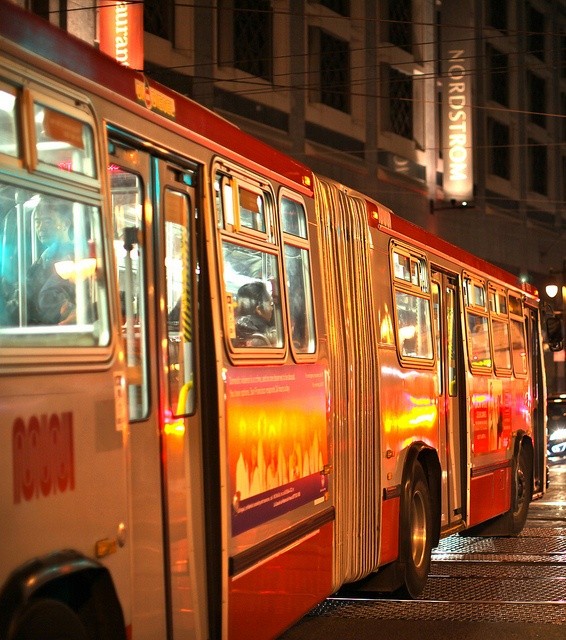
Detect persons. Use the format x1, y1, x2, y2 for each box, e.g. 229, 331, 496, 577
237, 280, 275, 347
19, 194, 76, 324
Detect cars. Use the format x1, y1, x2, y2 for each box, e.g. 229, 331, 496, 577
547, 397, 566, 466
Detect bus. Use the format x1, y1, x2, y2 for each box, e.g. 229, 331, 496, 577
1, 2, 547, 639
